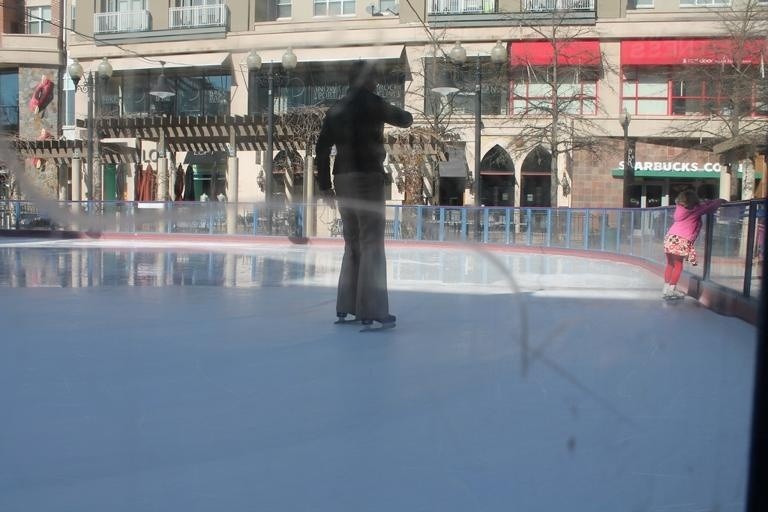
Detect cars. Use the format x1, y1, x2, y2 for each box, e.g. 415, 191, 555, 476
10, 214, 60, 231
650, 198, 747, 255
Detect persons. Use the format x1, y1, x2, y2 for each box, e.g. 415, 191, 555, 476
757, 202, 767, 278
314, 61, 414, 332
662, 188, 728, 295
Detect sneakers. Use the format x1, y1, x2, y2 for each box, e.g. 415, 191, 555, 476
337, 309, 397, 326
661, 286, 685, 299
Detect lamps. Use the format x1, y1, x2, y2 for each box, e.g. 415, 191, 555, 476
431, 54, 460, 97
149, 62, 177, 102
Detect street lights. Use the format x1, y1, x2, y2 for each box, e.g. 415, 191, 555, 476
617, 104, 635, 241
245, 46, 300, 236
447, 37, 513, 241
68, 55, 114, 215
